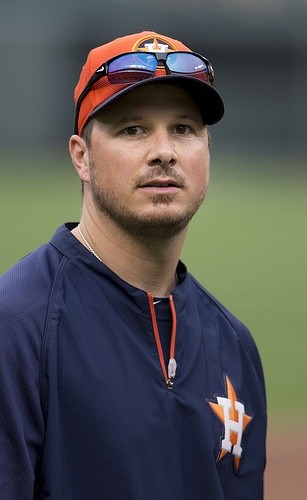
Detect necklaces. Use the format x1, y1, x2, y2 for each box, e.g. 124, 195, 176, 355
77, 223, 178, 292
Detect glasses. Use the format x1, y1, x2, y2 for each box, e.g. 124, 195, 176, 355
73, 50, 216, 135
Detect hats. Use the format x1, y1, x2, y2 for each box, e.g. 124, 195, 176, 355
73, 30, 226, 140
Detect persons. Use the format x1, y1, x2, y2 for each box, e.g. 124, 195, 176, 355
1, 31, 266, 500
